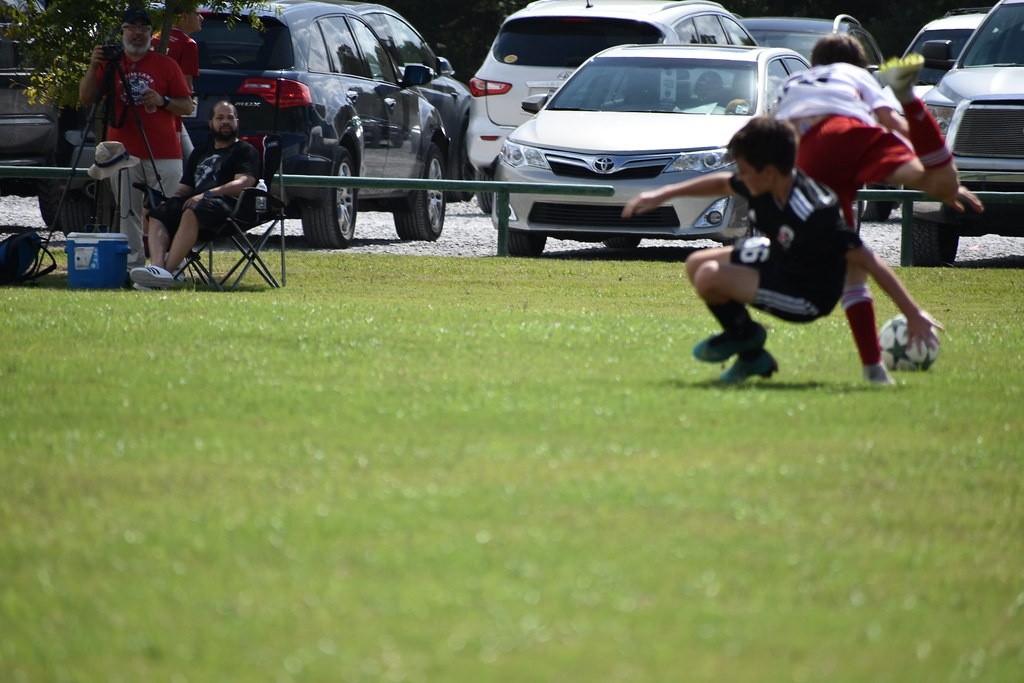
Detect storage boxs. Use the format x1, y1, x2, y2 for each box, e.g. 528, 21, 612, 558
61, 231, 129, 286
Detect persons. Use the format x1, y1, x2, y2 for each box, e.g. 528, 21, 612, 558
695, 71, 724, 103
772, 34, 983, 386
623, 118, 946, 382
79, 10, 194, 282
142, 0, 204, 255
130, 101, 260, 292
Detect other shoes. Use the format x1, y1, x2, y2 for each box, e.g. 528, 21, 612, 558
172, 266, 184, 281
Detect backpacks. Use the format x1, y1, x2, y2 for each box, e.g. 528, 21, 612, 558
0, 227, 56, 282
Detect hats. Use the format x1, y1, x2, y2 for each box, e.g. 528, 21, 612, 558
87, 141, 140, 181
122, 7, 152, 24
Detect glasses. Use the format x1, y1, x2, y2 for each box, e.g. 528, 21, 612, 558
122, 25, 151, 31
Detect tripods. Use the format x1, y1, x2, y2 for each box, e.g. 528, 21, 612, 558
28, 62, 197, 289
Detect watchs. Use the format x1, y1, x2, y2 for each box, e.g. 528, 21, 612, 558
204, 191, 211, 199
160, 95, 170, 109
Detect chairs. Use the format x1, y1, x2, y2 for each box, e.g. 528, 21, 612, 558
134, 133, 285, 292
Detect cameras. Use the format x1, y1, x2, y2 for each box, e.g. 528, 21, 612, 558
98, 45, 123, 62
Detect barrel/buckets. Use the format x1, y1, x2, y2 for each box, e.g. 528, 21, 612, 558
65, 232, 131, 289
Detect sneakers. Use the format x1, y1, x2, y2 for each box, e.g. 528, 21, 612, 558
133, 282, 166, 291
863, 364, 892, 384
721, 350, 774, 382
693, 323, 766, 361
130, 266, 174, 288
880, 53, 926, 88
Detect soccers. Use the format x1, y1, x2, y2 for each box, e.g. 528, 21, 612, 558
878, 313, 938, 370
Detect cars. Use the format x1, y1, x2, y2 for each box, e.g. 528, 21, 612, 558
492, 42, 812, 260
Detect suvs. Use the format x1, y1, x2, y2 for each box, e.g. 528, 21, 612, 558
728, 12, 885, 78
892, 0, 1024, 268
465, 1, 765, 218
0, 0, 470, 251
